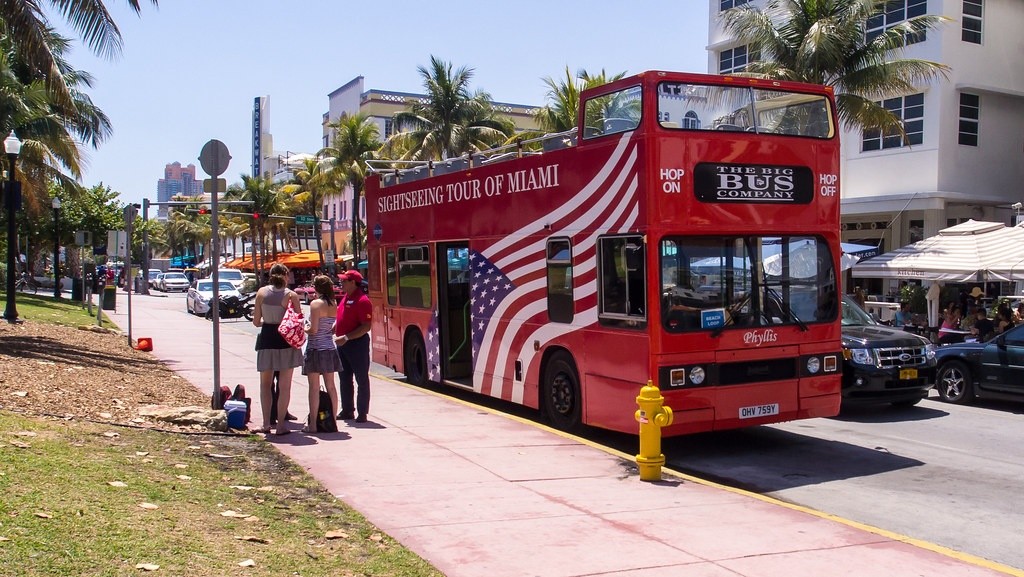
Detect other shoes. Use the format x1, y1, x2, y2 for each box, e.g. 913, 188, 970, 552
356, 414, 367, 423
276, 426, 290, 434
269, 422, 277, 428
302, 426, 317, 433
336, 411, 355, 420
285, 412, 297, 421
250, 426, 269, 433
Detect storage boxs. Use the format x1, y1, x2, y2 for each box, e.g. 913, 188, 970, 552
223, 399, 247, 430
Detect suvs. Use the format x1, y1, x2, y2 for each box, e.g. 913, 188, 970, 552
696, 284, 939, 415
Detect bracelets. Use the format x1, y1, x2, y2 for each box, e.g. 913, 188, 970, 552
344, 335, 349, 341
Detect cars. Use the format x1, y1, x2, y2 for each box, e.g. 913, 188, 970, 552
209, 269, 249, 289
139, 269, 191, 292
927, 322, 1024, 405
186, 279, 243, 316
294, 283, 344, 305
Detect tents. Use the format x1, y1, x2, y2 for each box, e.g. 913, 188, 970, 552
690, 240, 879, 279
851, 219, 1024, 326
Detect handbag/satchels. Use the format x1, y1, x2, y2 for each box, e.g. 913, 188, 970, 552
307, 384, 338, 433
212, 384, 251, 422
278, 293, 306, 349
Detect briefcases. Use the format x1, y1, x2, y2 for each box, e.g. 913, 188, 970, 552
224, 399, 247, 428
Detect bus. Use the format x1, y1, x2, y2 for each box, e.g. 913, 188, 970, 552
362, 69, 843, 433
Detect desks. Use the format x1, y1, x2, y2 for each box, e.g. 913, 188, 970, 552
938, 329, 972, 343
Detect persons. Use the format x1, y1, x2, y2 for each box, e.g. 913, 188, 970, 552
94, 266, 125, 290
251, 264, 344, 435
331, 270, 372, 422
550, 239, 643, 329
893, 281, 1024, 347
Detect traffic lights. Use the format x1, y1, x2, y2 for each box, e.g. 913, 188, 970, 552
253, 213, 268, 219
198, 210, 211, 214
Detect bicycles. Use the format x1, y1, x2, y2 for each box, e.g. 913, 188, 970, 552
14, 272, 37, 295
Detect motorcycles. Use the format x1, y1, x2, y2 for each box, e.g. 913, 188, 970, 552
205, 292, 257, 322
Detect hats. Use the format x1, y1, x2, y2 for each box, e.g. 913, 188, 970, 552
969, 287, 985, 297
337, 270, 362, 284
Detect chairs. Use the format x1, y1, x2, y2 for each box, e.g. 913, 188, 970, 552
383, 118, 773, 189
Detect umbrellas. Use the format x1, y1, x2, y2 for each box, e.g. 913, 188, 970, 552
194, 250, 343, 271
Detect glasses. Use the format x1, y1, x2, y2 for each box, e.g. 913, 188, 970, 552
339, 279, 346, 283
